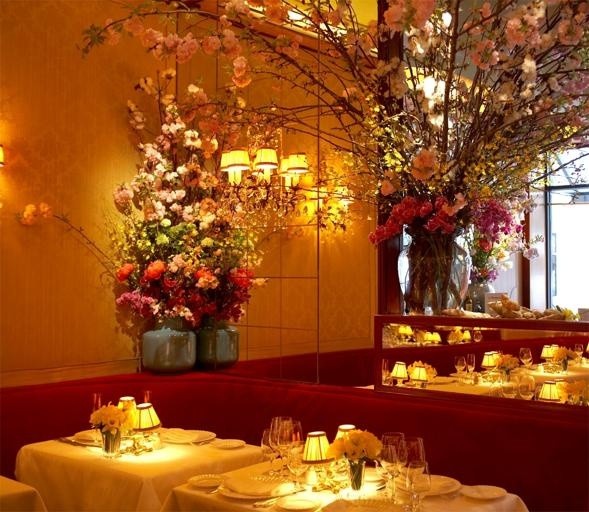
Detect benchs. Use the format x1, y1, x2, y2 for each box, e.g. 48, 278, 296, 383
0, 334, 589, 511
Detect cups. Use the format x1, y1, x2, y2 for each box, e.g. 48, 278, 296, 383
473, 331, 482, 342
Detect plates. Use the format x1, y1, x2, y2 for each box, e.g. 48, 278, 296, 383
189, 473, 405, 512
386, 474, 507, 499
432, 372, 481, 384
74, 428, 245, 449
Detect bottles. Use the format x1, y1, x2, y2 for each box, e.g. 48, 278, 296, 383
424, 307, 434, 316
404, 335, 416, 342
464, 296, 482, 312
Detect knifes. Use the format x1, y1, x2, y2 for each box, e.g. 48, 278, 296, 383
58, 438, 86, 447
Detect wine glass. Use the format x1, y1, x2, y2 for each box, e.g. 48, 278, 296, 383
490, 375, 535, 400
379, 432, 431, 512
519, 344, 584, 372
261, 417, 311, 489
456, 353, 475, 386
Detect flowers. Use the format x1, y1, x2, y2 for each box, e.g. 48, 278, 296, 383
19, 0, 589, 320
